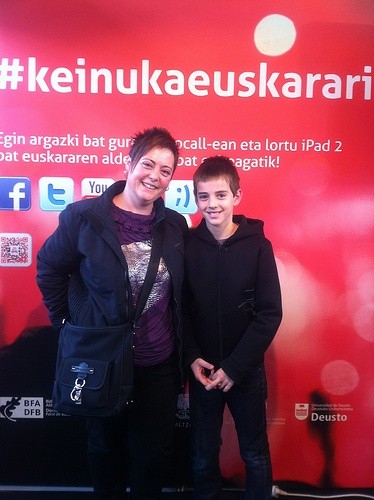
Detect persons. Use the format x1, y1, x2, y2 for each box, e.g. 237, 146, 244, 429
34, 128, 190, 500
182, 155, 283, 500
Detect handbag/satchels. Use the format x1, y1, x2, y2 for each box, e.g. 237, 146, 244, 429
53, 321, 135, 419
